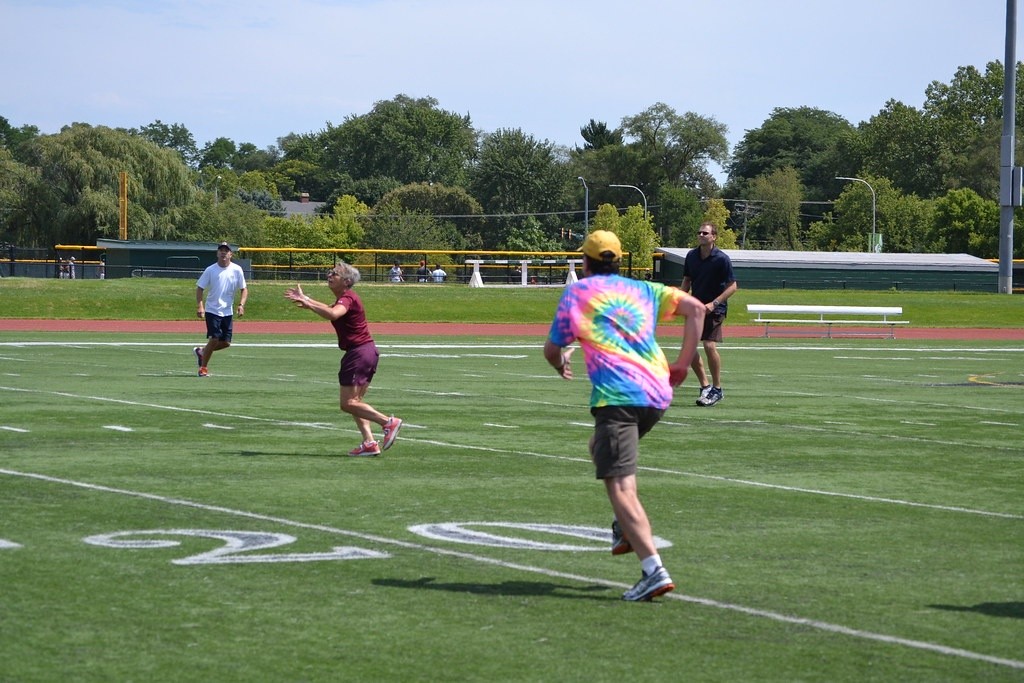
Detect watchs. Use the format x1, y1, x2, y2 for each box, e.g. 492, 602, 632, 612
713, 299, 719, 306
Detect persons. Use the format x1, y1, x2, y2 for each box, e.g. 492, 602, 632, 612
416, 260, 432, 283
511, 267, 522, 284
96, 259, 105, 279
55, 257, 76, 279
432, 264, 447, 283
544, 230, 706, 601
645, 273, 652, 281
388, 260, 406, 283
562, 268, 578, 285
680, 223, 738, 406
531, 277, 537, 285
283, 262, 403, 456
193, 242, 248, 378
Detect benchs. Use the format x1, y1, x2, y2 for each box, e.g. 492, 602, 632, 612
747, 304, 909, 340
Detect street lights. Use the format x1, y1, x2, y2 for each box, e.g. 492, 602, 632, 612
835, 177, 875, 253
215, 175, 225, 197
609, 185, 647, 220
577, 177, 590, 235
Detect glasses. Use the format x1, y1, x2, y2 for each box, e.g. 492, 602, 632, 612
697, 231, 713, 236
326, 269, 344, 278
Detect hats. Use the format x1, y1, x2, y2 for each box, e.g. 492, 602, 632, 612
216, 241, 232, 250
583, 231, 623, 263
420, 260, 424, 265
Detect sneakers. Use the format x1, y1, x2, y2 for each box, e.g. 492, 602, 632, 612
622, 566, 675, 602
347, 441, 381, 457
697, 384, 712, 403
198, 367, 208, 377
612, 520, 633, 555
195, 347, 204, 369
700, 387, 723, 406
382, 414, 402, 450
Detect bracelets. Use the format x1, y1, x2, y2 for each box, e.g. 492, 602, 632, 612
305, 296, 309, 301
555, 354, 566, 370
238, 304, 244, 307
